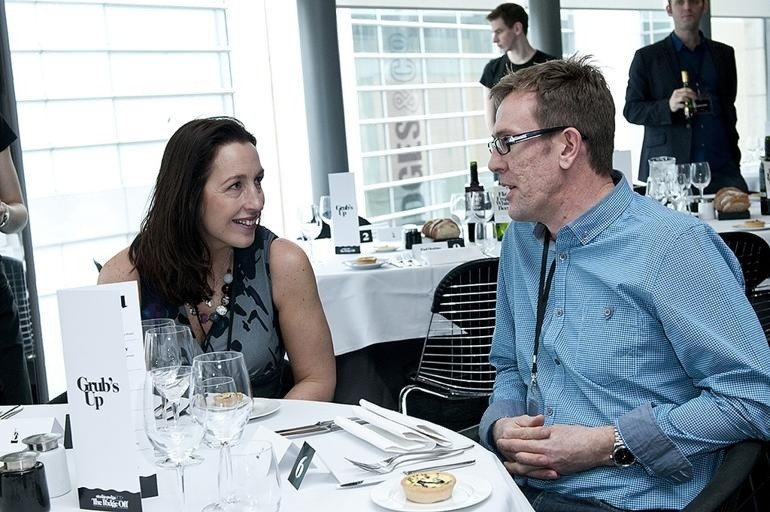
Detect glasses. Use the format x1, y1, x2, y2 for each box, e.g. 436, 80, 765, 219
485, 124, 574, 155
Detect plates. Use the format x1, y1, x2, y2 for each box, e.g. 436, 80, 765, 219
341, 224, 465, 270
729, 222, 770, 232
370, 473, 493, 512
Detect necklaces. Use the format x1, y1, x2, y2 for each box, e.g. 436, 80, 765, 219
189, 252, 234, 320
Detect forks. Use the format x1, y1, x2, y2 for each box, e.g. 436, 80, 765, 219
358, 450, 464, 473
344, 444, 479, 468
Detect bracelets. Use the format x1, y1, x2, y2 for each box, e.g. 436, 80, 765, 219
0, 204, 10, 230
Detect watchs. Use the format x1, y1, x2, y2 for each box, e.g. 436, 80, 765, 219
610, 426, 636, 470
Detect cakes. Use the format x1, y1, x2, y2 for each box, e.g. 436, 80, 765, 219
356, 255, 376, 265
213, 393, 243, 407
746, 220, 765, 228
401, 471, 456, 505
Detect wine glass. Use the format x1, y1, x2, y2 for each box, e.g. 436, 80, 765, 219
135, 315, 282, 512
319, 196, 333, 245
450, 159, 509, 259
297, 203, 322, 259
644, 155, 713, 223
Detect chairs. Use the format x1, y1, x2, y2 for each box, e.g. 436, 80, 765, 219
674, 436, 769, 510
306, 212, 372, 238
396, 250, 501, 432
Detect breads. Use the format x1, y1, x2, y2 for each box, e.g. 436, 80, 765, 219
422, 218, 461, 239
714, 186, 751, 213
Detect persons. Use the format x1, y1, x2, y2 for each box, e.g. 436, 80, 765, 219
622, 0, 748, 196
478, 4, 561, 130
478, 51, 769, 511
0, 114, 33, 404
97, 116, 338, 408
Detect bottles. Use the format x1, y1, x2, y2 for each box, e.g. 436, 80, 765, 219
18, 431, 73, 500
757, 135, 770, 217
697, 198, 716, 222
680, 70, 704, 131
0, 450, 52, 511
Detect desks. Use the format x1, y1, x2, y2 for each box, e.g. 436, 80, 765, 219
273, 219, 770, 362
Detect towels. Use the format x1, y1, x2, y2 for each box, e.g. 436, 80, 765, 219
334, 398, 454, 455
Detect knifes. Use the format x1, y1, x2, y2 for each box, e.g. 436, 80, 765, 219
335, 460, 477, 491
279, 421, 369, 438
271, 416, 360, 434
